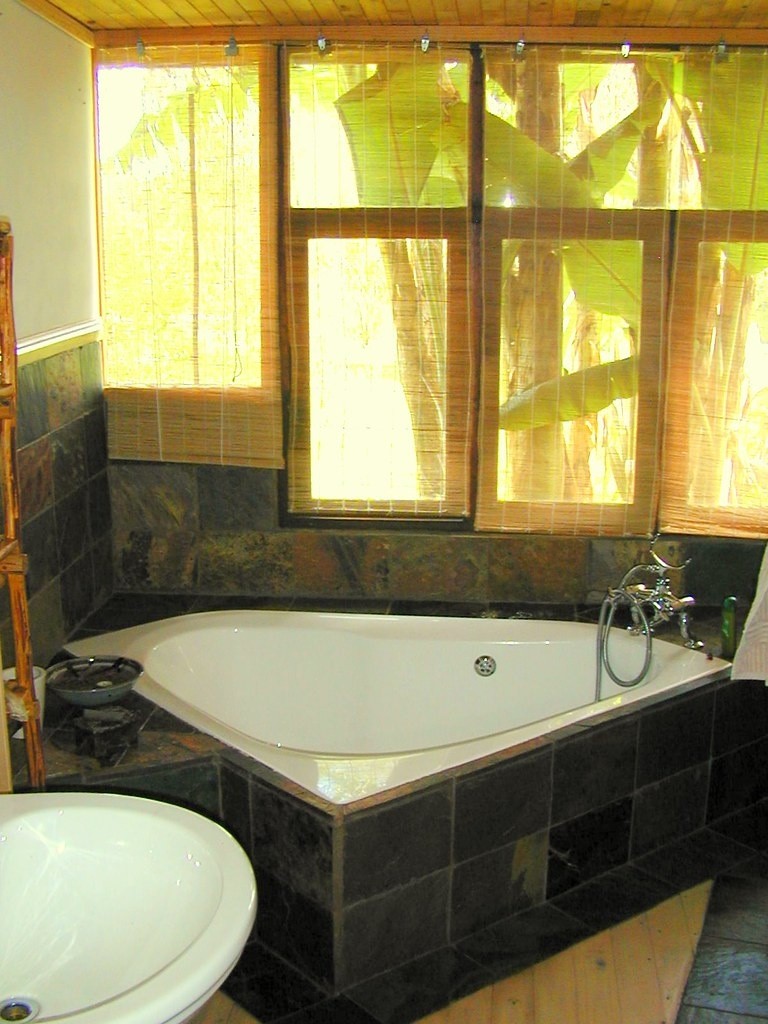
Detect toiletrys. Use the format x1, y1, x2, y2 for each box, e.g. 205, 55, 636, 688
720, 595, 737, 658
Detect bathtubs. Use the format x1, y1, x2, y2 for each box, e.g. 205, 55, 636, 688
58, 603, 740, 809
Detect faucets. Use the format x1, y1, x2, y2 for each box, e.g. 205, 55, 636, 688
626, 614, 662, 637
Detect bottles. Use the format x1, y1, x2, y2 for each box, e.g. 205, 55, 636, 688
720, 596, 738, 658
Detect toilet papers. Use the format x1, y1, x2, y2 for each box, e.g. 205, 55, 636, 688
3, 664, 48, 739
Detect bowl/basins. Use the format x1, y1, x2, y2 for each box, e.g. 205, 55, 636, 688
45, 654, 145, 705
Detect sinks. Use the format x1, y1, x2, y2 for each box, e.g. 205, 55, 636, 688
0, 788, 257, 1023
